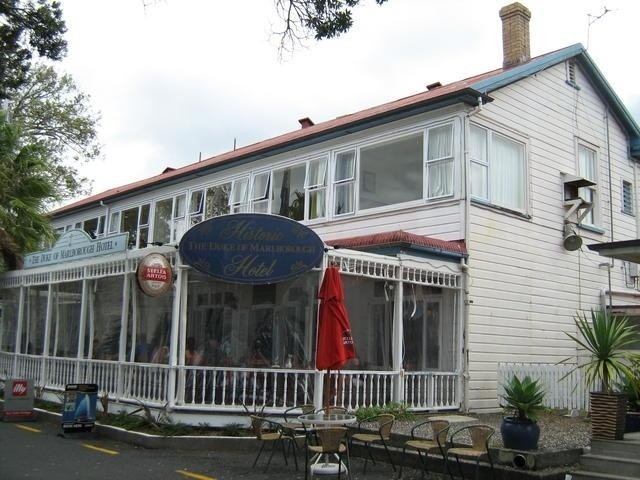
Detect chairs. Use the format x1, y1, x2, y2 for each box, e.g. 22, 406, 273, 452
315, 406, 347, 415
351, 414, 398, 474
304, 426, 354, 480
249, 414, 298, 474
281, 404, 317, 459
399, 418, 455, 479
445, 423, 498, 479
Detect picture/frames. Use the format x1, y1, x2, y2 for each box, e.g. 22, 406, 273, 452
362, 170, 377, 193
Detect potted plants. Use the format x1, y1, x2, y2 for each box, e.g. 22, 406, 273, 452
498, 373, 547, 451
553, 300, 639, 444
610, 358, 639, 433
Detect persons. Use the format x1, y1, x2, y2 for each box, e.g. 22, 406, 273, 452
136, 334, 152, 362
186, 337, 202, 365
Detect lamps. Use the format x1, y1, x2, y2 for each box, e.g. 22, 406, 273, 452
561, 220, 583, 252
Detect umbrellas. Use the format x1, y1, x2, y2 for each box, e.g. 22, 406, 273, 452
308, 264, 357, 467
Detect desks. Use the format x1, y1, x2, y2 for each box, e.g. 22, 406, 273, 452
298, 412, 357, 476
